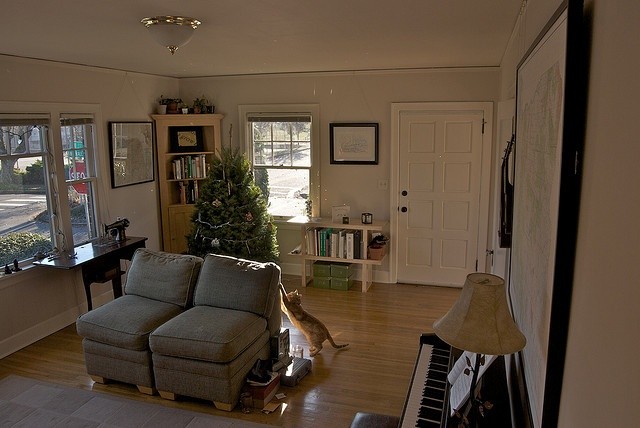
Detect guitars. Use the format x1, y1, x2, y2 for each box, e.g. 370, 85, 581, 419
498, 134, 515, 248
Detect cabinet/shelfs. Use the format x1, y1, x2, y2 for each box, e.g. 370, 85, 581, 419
284, 211, 390, 294
150, 113, 225, 253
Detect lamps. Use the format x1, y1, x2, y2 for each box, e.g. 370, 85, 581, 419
139, 14, 202, 58
432, 272, 527, 422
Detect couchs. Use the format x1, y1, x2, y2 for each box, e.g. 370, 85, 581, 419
148, 252, 281, 412
75, 247, 204, 396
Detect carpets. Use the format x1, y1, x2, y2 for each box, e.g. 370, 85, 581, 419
0, 373, 278, 428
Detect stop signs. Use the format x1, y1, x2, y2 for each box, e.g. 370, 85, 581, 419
69, 161, 89, 195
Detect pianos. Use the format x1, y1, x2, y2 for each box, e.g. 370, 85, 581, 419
397, 333, 526, 428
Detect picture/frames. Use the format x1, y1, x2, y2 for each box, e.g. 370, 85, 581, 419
107, 121, 155, 189
329, 122, 379, 165
505, 0, 591, 428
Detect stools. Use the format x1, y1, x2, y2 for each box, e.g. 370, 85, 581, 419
347, 411, 400, 428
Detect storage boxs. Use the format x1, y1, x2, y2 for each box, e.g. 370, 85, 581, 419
331, 272, 356, 291
241, 372, 281, 410
330, 264, 352, 278
313, 276, 331, 289
312, 260, 331, 277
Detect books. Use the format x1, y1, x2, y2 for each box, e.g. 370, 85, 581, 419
171, 155, 211, 179
305, 229, 363, 260
179, 181, 198, 205
447, 351, 498, 417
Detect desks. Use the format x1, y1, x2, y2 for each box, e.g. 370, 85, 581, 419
31, 236, 148, 269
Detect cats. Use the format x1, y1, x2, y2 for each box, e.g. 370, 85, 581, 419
279, 283, 349, 357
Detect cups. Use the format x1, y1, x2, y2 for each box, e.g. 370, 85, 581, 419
240, 392, 253, 414
292, 345, 303, 358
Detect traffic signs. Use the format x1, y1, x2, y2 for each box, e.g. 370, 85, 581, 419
69, 141, 85, 156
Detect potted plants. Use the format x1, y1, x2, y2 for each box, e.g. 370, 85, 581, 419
373, 234, 390, 254
190, 96, 209, 114
179, 102, 189, 114
368, 239, 383, 259
158, 97, 183, 114
157, 94, 167, 115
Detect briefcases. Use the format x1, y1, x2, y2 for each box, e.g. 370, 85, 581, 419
279, 355, 312, 385
